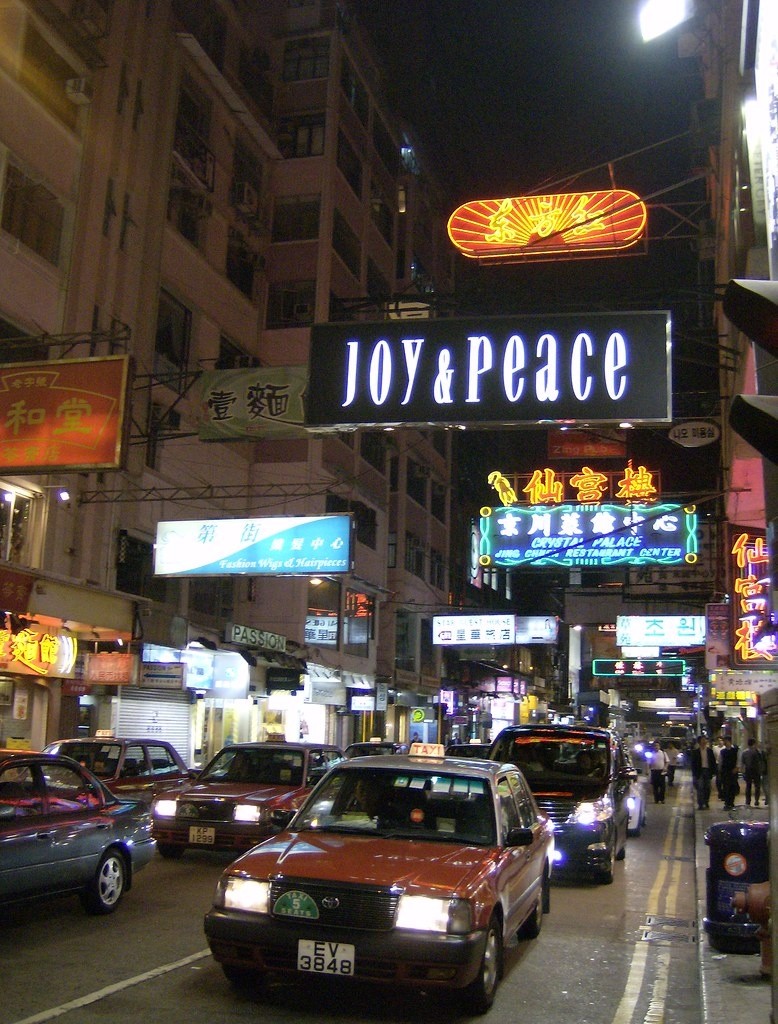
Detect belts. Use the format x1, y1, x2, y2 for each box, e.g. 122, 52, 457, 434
651, 770, 662, 771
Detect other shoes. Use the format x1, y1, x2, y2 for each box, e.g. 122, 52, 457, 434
746, 801, 750, 805
765, 801, 768, 805
722, 805, 731, 811
655, 801, 658, 804
754, 801, 759, 806
705, 803, 709, 808
718, 794, 722, 800
661, 801, 665, 804
698, 804, 703, 810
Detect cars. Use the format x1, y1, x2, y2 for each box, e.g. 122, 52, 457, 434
202, 742, 557, 1017
313, 737, 411, 789
653, 738, 688, 768
445, 739, 497, 763
148, 732, 350, 859
13, 729, 189, 819
0, 750, 160, 918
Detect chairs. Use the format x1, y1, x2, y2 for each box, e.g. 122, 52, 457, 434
456, 801, 491, 832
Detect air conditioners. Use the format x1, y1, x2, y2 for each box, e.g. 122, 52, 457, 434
64, 77, 95, 105
294, 303, 314, 319
233, 182, 258, 216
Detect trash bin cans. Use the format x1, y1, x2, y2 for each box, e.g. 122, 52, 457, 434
703, 818, 772, 955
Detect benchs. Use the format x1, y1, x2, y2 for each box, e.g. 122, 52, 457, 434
114, 758, 165, 769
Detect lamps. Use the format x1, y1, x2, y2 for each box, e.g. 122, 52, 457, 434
43, 485, 70, 500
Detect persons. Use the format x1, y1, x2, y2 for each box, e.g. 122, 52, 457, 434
92, 760, 106, 771
692, 735, 769, 811
410, 732, 423, 748
649, 740, 678, 804
575, 750, 597, 777
348, 779, 375, 812
440, 733, 471, 746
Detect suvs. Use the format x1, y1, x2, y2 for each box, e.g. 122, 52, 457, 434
480, 723, 642, 884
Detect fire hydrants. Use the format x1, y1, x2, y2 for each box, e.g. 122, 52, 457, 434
731, 880, 774, 980
